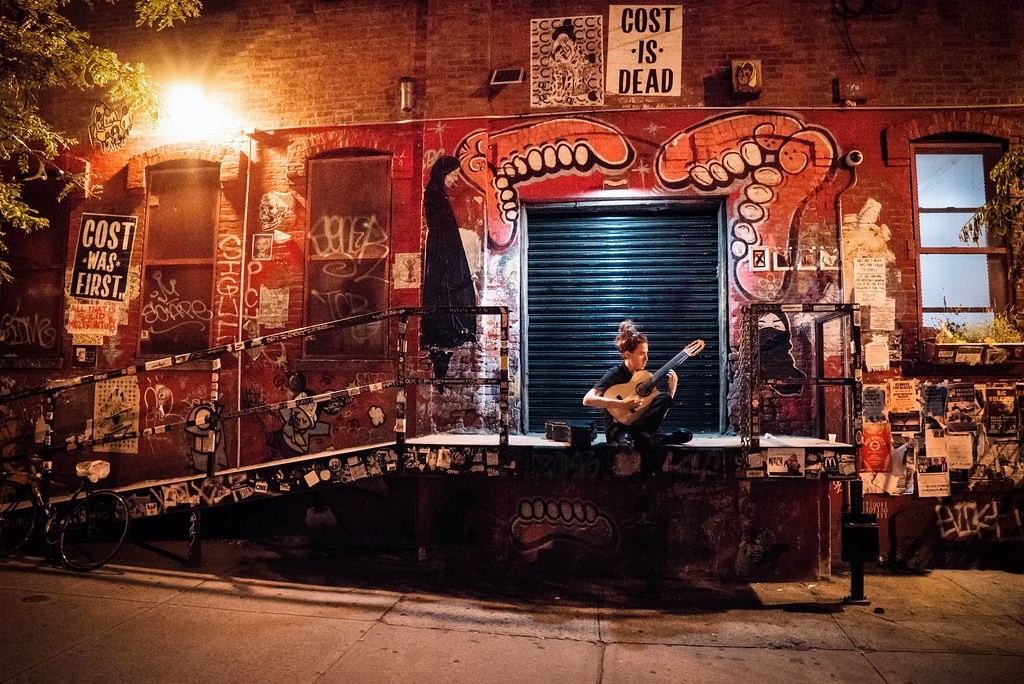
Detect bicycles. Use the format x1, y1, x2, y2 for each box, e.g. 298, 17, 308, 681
0, 455, 130, 570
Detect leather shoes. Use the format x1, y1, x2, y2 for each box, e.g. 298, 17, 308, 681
615, 432, 635, 453
654, 427, 694, 445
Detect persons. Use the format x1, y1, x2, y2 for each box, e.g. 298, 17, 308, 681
582, 319, 693, 453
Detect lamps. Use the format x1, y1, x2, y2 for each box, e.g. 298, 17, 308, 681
400, 76, 416, 113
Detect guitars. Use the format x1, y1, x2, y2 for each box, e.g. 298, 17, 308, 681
601, 338, 706, 425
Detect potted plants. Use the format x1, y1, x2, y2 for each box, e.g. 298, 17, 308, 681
919, 296, 1024, 365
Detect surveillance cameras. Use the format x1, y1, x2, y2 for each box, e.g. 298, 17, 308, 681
846, 150, 863, 167
